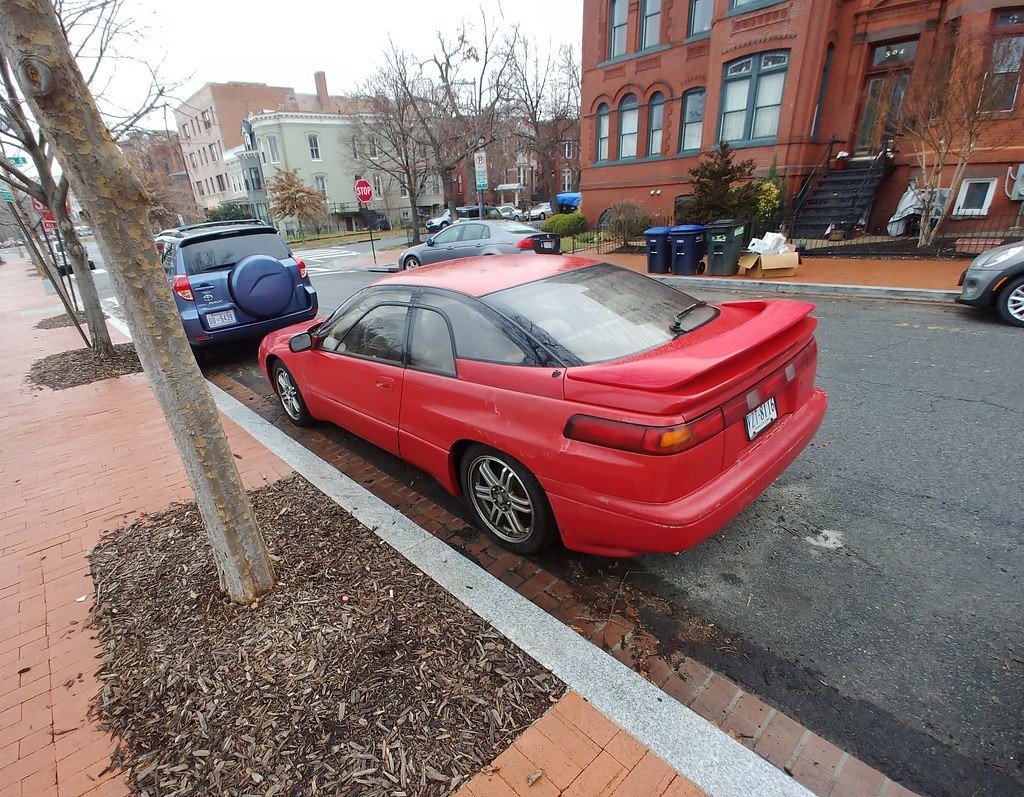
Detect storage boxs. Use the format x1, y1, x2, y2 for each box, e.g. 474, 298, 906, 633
737, 244, 802, 279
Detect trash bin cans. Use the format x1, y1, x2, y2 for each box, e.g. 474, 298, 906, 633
670, 224, 706, 276
704, 219, 747, 276
643, 226, 671, 274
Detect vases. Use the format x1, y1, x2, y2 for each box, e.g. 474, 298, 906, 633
829, 230, 845, 241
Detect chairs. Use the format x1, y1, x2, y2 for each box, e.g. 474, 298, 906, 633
421, 303, 473, 368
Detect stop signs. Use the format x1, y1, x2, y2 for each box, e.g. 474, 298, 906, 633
354, 180, 373, 201
31, 192, 71, 221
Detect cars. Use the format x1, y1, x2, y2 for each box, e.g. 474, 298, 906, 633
48, 240, 96, 277
398, 219, 563, 270
258, 253, 828, 556
955, 238, 1024, 328
490, 206, 523, 222
425, 207, 469, 230
523, 203, 554, 221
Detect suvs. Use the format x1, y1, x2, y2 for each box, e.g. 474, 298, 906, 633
153, 219, 319, 347
